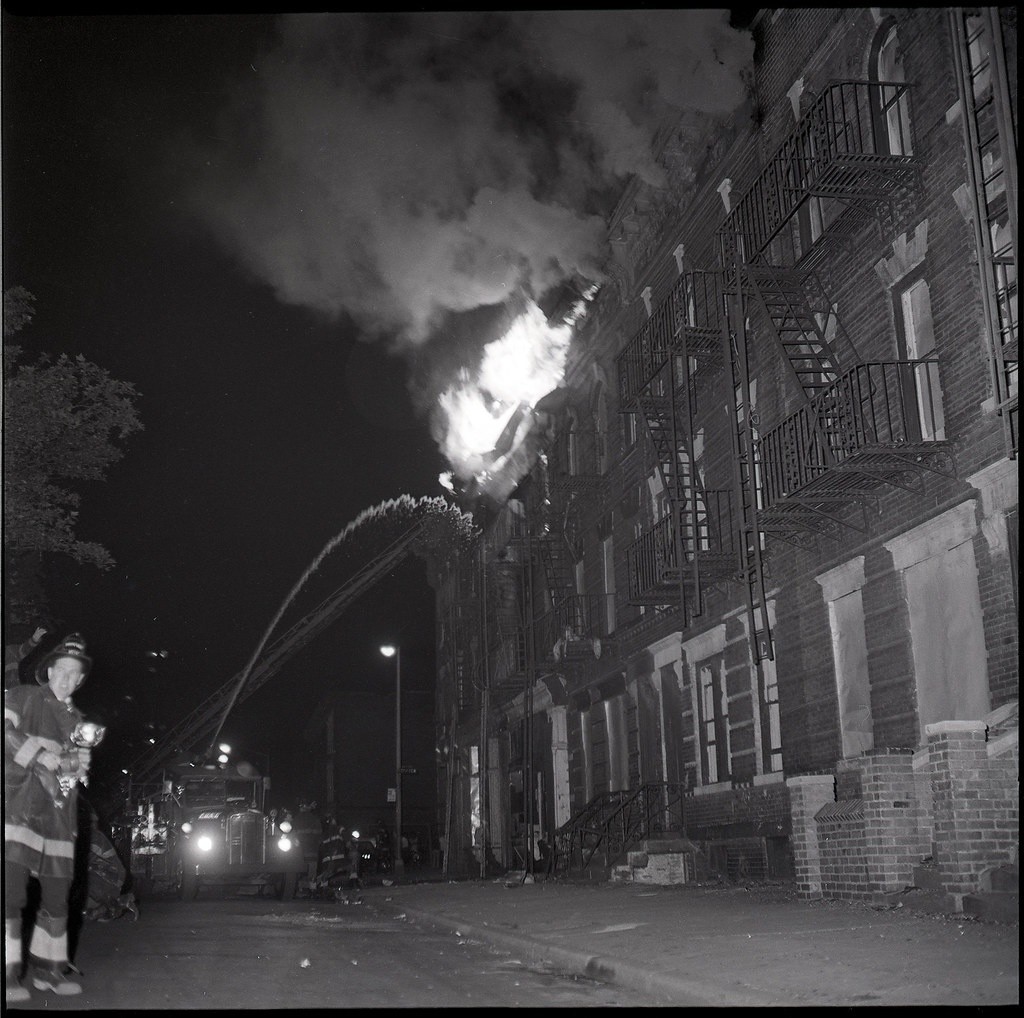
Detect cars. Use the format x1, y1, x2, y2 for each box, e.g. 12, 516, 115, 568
353, 838, 407, 886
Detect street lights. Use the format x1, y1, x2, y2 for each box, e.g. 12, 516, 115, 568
122, 769, 132, 810
380, 635, 401, 865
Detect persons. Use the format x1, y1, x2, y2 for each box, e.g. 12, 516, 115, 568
359, 824, 419, 875
273, 800, 365, 903
5, 627, 139, 1001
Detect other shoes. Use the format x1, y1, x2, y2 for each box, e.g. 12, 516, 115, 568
6, 967, 83, 1000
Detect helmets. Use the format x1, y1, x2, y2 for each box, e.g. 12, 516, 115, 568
324, 814, 335, 824
277, 807, 290, 821
36, 632, 93, 689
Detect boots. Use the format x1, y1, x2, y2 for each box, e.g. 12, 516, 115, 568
351, 875, 360, 891
321, 876, 329, 887
308, 877, 318, 890
97, 890, 141, 924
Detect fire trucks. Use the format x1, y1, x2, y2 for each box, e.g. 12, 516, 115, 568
111, 742, 307, 903
